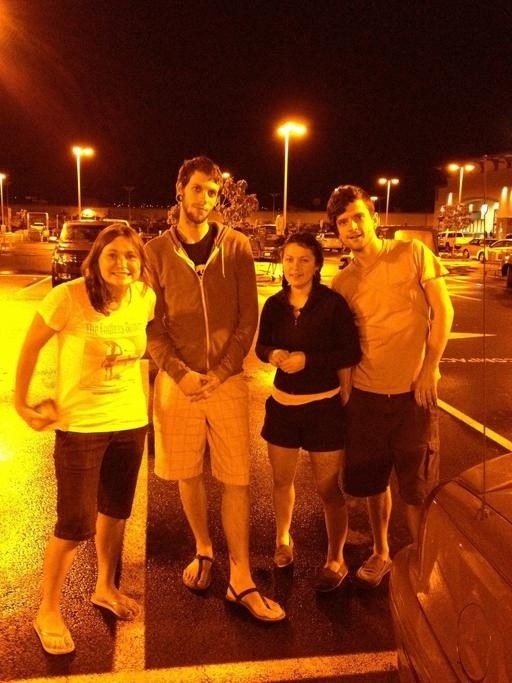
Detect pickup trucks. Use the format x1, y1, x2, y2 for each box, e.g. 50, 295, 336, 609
437, 230, 473, 250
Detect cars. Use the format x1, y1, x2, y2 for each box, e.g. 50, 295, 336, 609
500, 254, 512, 286
315, 232, 347, 251
460, 238, 497, 259
250, 223, 284, 262
388, 450, 510, 682
31, 223, 45, 230
503, 233, 512, 239
476, 238, 512, 262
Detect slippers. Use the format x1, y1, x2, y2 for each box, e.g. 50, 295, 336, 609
90, 594, 141, 621
32, 619, 75, 655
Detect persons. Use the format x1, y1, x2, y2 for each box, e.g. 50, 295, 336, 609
145, 155, 288, 624
252, 232, 356, 594
326, 183, 459, 586
12, 220, 160, 655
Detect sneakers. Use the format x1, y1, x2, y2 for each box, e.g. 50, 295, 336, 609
274, 532, 294, 569
356, 554, 392, 586
312, 561, 348, 592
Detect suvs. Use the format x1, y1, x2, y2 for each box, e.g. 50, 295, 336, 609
48, 218, 130, 290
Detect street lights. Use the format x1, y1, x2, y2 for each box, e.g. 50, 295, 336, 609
447, 162, 476, 207
0, 173, 7, 224
72, 146, 95, 215
274, 121, 307, 230
377, 176, 402, 225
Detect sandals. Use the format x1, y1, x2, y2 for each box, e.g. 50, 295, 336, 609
183, 554, 214, 591
224, 582, 286, 622
